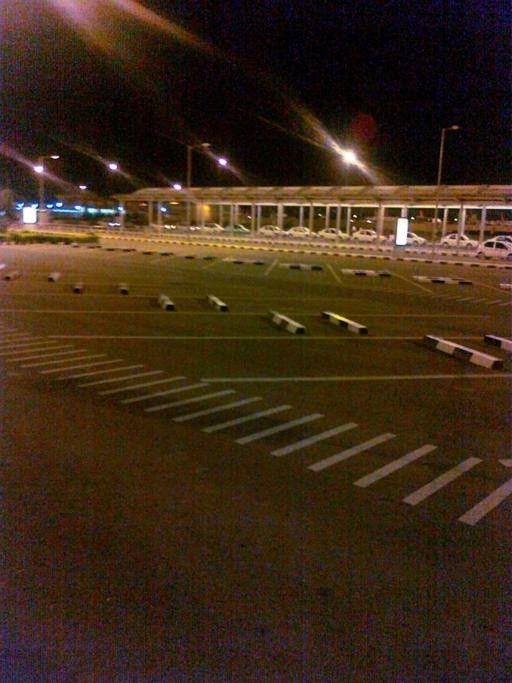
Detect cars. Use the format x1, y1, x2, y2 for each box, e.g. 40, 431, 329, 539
286, 226, 317, 241
159, 223, 177, 232
259, 225, 287, 238
352, 228, 388, 244
203, 223, 223, 231
440, 232, 480, 250
105, 220, 120, 229
317, 227, 350, 242
388, 231, 428, 248
476, 234, 512, 260
188, 224, 202, 232
224, 223, 250, 234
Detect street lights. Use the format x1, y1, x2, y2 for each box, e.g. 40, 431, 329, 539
183, 142, 211, 226
434, 125, 460, 222
37, 154, 60, 220
